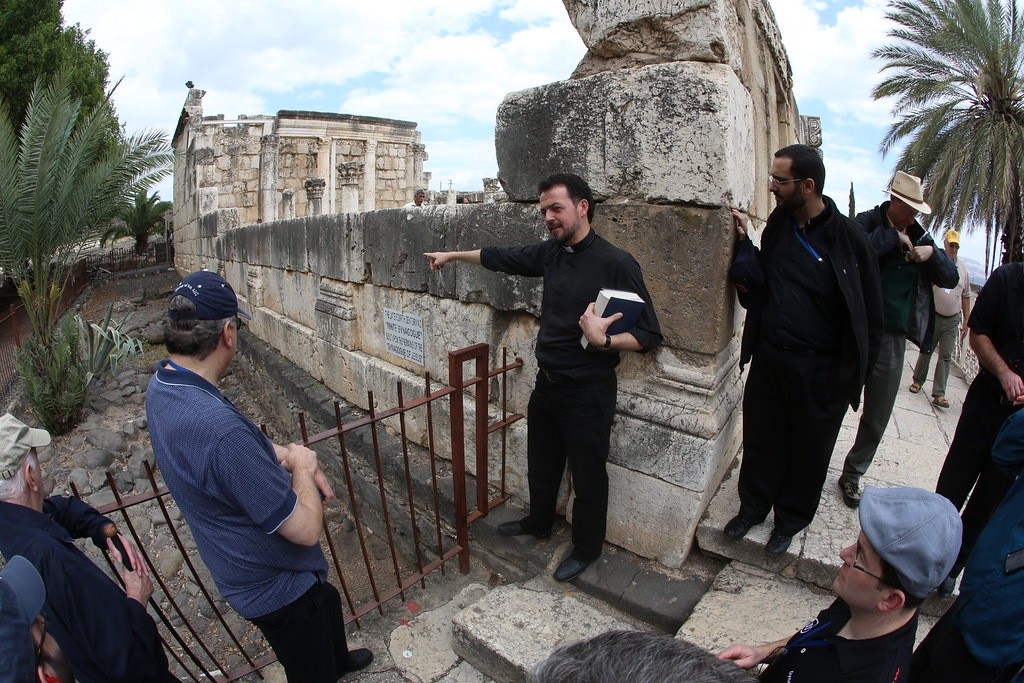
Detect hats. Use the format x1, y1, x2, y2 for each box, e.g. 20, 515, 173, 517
859, 485, 963, 599
945, 230, 960, 245
0, 413, 51, 481
0, 555, 46, 683
882, 170, 932, 215
168, 269, 253, 321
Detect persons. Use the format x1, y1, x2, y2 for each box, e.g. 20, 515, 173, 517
721, 144, 885, 558
715, 484, 964, 683
147, 272, 373, 682
936, 262, 1024, 598
525, 628, 759, 683
405, 189, 425, 207
908, 228, 971, 408
838, 168, 959, 508
420, 172, 663, 582
907, 408, 1024, 683
0, 555, 77, 683
0, 413, 185, 683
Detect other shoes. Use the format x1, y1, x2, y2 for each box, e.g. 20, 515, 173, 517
838, 475, 860, 508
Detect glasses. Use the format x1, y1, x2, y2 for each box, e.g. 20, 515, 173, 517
746, 646, 789, 681
768, 175, 803, 187
229, 318, 246, 331
853, 538, 910, 609
946, 240, 959, 249
36, 610, 48, 670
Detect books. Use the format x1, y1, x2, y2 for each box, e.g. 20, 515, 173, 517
580, 288, 646, 351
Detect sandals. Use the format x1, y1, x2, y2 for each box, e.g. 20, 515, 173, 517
932, 395, 949, 408
909, 382, 922, 393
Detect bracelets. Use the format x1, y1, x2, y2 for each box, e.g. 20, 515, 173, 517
603, 335, 611, 350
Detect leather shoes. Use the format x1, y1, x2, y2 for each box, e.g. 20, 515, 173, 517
764, 529, 793, 559
552, 555, 593, 582
496, 520, 527, 535
938, 575, 956, 599
723, 515, 761, 540
336, 648, 374, 679
